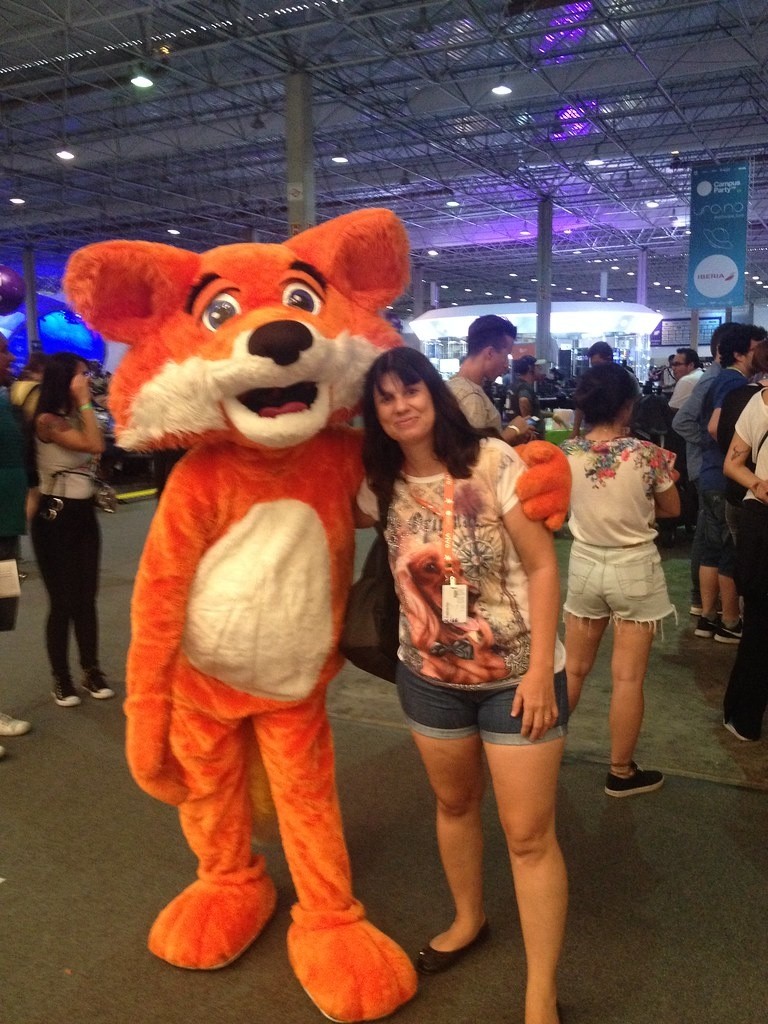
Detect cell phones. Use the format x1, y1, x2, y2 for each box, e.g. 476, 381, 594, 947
527, 416, 541, 424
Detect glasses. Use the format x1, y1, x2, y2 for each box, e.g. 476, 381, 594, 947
670, 361, 688, 366
74, 371, 95, 377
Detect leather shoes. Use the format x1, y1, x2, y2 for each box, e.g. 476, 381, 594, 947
413, 909, 488, 975
556, 999, 563, 1024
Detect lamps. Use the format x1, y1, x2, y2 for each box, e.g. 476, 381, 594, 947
6, 6, 681, 255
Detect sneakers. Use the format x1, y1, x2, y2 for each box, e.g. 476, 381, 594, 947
690, 615, 743, 644
81, 667, 115, 699
0, 711, 31, 737
50, 673, 82, 707
604, 760, 665, 798
0, 746, 5, 757
689, 595, 741, 615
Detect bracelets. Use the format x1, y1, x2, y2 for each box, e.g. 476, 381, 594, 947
509, 424, 521, 435
77, 401, 94, 412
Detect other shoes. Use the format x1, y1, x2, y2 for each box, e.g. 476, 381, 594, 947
722, 714, 753, 742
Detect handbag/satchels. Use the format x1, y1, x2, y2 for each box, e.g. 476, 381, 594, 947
725, 430, 768, 507
12, 383, 43, 427
338, 476, 401, 685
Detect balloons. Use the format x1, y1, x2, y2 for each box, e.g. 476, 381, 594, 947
0, 264, 27, 316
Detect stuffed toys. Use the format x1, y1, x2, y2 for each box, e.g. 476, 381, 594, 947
60, 205, 574, 1024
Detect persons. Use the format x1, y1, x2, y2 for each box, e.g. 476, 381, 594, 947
0, 313, 768, 760
353, 345, 570, 1024
561, 362, 681, 796
